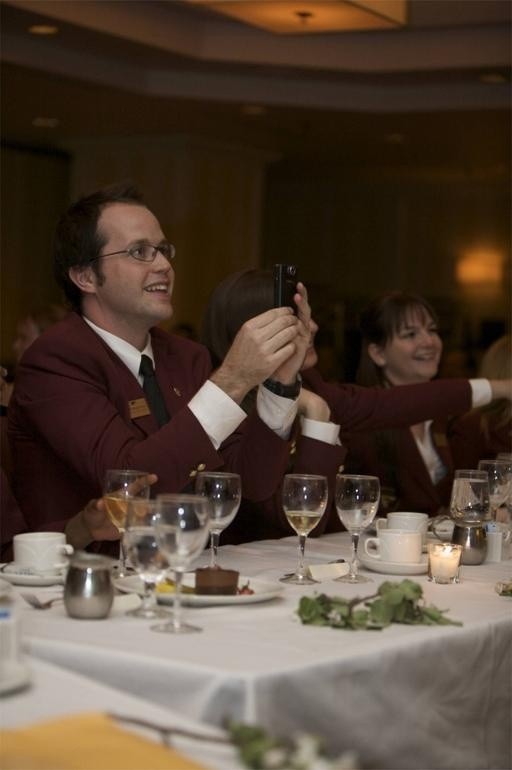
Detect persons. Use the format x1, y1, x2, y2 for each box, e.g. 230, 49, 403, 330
0, 303, 71, 466
0, 464, 159, 564
206, 267, 512, 548
172, 323, 198, 342
450, 333, 512, 468
327, 290, 512, 534
8, 186, 311, 565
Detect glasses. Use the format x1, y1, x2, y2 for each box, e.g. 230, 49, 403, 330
83, 241, 178, 264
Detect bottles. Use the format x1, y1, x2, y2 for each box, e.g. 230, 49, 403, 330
62, 552, 114, 621
450, 469, 492, 525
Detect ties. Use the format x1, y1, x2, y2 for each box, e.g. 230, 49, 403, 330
138, 353, 171, 430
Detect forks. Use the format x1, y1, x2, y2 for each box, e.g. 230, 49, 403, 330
19, 591, 65, 611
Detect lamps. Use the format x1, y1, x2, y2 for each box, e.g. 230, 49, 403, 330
211, 0, 408, 36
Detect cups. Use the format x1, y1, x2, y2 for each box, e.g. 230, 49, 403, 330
376, 511, 428, 546
1, 610, 18, 670
362, 527, 425, 565
13, 532, 75, 575
424, 540, 464, 585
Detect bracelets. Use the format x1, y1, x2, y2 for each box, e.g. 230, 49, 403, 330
263, 374, 302, 398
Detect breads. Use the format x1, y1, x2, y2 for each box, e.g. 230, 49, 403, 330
195, 568, 240, 596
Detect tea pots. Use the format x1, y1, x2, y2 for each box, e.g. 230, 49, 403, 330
432, 516, 487, 566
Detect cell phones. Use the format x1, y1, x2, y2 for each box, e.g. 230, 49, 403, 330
275, 263, 299, 313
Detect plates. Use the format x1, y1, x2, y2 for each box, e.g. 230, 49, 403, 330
356, 554, 432, 577
109, 569, 293, 607
0, 662, 38, 698
422, 537, 444, 554
0, 562, 70, 587
425, 516, 454, 534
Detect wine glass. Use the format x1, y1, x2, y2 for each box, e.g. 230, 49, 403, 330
494, 453, 511, 544
102, 464, 210, 633
331, 472, 382, 584
478, 459, 512, 534
192, 471, 243, 569
277, 472, 328, 586
